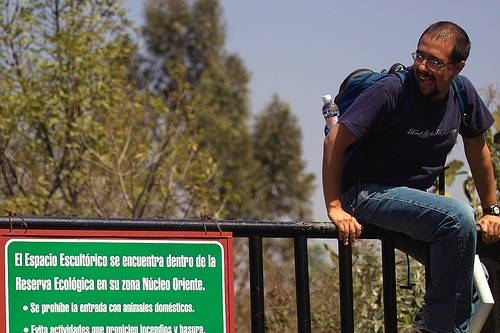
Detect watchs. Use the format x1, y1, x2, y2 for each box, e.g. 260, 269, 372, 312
481, 203, 500, 216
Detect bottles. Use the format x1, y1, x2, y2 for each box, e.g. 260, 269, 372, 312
322, 95, 340, 130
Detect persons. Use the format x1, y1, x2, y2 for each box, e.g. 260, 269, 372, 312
321, 21, 500, 333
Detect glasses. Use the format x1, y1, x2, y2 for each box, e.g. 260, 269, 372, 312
410, 52, 454, 68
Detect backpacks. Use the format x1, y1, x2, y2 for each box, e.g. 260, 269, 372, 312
324, 64, 471, 138
